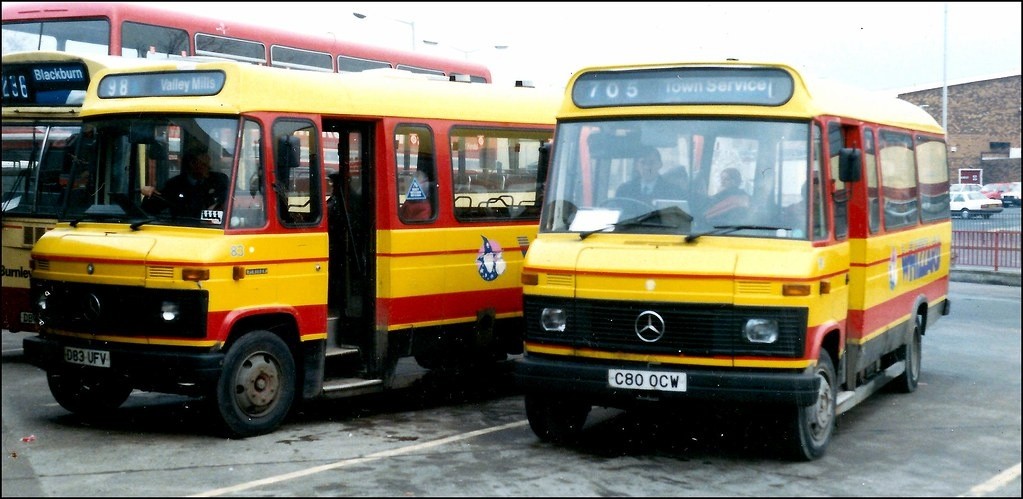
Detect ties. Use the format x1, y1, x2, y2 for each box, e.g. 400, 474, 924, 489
642, 187, 648, 197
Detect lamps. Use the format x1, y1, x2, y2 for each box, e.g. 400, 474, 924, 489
951, 144, 959, 153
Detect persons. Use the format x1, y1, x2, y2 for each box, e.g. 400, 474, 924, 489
142, 143, 229, 223
709, 168, 752, 205
612, 147, 687, 226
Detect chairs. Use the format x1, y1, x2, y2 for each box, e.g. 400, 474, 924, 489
455, 194, 538, 219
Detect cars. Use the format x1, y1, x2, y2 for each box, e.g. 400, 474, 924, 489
978, 183, 1017, 200
921, 191, 1004, 220
950, 184, 982, 191
1001, 184, 1021, 208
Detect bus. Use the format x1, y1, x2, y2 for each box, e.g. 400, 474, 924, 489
0, 55, 546, 334
21, 60, 704, 441
510, 60, 952, 463
1, 2, 498, 201
